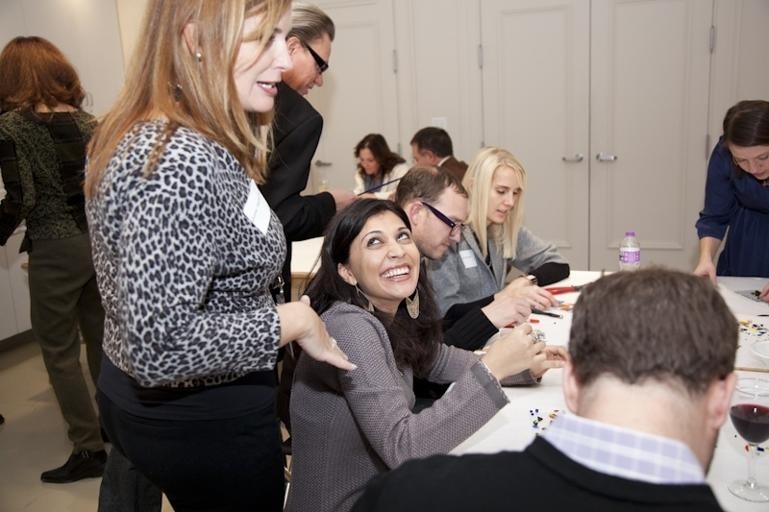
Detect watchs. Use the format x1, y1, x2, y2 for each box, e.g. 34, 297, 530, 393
525, 274, 541, 286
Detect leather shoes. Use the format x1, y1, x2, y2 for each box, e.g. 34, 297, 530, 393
40, 450, 108, 484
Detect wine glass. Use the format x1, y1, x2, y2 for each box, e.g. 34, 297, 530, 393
729, 377, 768, 504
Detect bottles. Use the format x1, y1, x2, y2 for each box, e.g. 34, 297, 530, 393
619, 230, 642, 274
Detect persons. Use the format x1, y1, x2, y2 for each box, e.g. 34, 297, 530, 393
79, 0, 297, 512
424, 145, 571, 333
352, 134, 406, 195
348, 260, 740, 511
276, 162, 532, 440
1, 34, 112, 483
409, 126, 468, 185
276, 195, 572, 511
690, 99, 769, 303
97, 1, 394, 511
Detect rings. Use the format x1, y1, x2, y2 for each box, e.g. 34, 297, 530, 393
531, 335, 539, 344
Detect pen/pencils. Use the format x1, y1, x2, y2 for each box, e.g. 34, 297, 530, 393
734, 367, 769, 372
532, 309, 560, 318
757, 315, 769, 316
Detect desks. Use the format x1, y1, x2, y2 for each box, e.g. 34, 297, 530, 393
445, 270, 769, 511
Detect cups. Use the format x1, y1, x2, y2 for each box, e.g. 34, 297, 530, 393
310, 164, 333, 195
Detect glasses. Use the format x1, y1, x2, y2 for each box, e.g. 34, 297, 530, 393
302, 39, 329, 75
422, 200, 465, 237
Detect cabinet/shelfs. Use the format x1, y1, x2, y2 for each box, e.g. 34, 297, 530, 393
292, 2, 398, 203
480, 0, 712, 276
